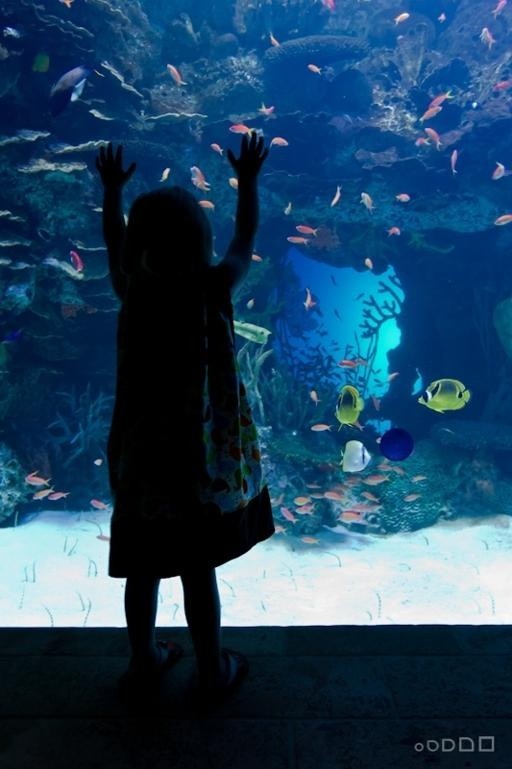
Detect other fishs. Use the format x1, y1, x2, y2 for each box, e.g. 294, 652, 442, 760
0, 1, 511, 546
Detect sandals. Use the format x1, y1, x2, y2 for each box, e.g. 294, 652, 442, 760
190, 648, 261, 692
118, 639, 185, 695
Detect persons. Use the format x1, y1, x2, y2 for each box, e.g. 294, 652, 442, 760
91, 128, 277, 706
